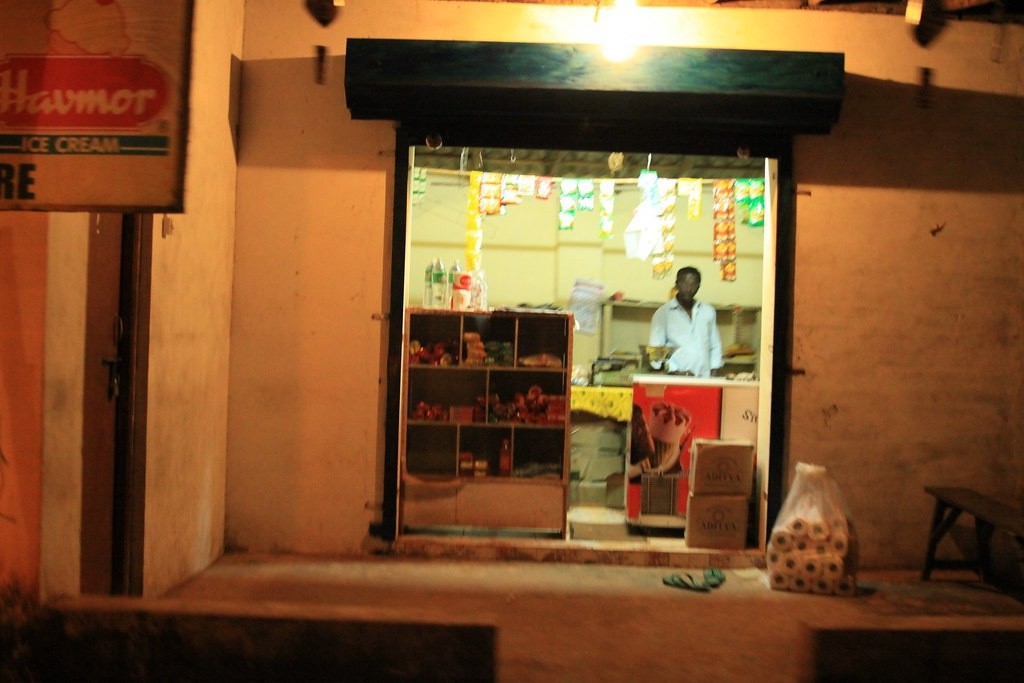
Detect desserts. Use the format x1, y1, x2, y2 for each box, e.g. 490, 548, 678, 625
649, 402, 691, 469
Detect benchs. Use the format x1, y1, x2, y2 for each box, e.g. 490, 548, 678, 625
921, 486, 1024, 598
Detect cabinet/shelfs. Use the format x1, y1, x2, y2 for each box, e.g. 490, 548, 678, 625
601, 299, 762, 377
625, 374, 759, 534
400, 309, 574, 540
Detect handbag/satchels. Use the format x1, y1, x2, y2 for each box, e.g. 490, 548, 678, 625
767, 463, 857, 597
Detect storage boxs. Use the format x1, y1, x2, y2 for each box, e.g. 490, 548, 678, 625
685, 437, 755, 550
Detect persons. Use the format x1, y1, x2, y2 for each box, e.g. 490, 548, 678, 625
646, 265, 728, 378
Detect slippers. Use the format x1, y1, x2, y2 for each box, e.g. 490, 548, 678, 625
662, 574, 708, 593
704, 568, 723, 588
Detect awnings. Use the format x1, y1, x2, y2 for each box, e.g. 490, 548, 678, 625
343, 33, 846, 136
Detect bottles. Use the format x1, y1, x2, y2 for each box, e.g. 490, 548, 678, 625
423, 259, 435, 306
432, 258, 446, 307
451, 261, 460, 306
500, 440, 510, 475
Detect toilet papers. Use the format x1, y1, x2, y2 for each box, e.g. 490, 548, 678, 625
766, 506, 855, 596
